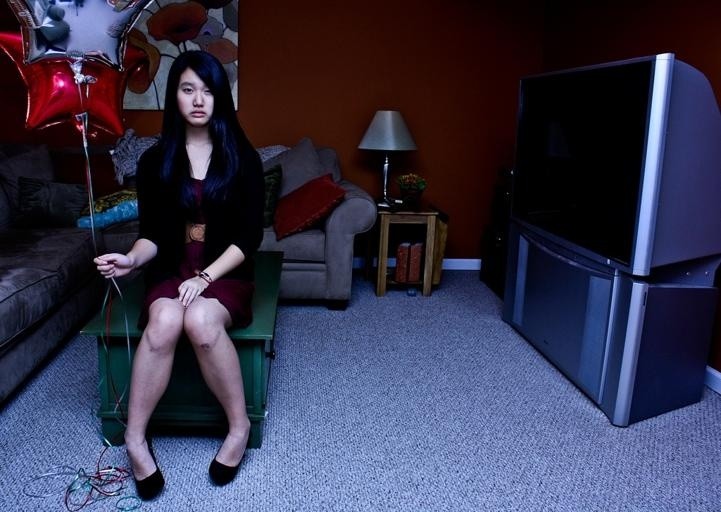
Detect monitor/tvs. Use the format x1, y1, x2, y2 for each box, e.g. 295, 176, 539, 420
517, 51, 721, 287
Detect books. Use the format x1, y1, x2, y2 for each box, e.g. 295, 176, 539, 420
395, 241, 410, 284
407, 241, 425, 282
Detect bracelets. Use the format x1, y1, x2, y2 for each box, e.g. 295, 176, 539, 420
193, 269, 212, 285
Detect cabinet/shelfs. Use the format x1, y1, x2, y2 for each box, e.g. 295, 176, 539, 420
501, 229, 720, 428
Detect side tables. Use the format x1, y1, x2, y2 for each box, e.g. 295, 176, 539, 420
375, 207, 440, 297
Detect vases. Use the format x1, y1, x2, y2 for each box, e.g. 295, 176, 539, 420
401, 189, 421, 212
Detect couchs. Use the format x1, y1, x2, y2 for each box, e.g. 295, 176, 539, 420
0, 145, 377, 409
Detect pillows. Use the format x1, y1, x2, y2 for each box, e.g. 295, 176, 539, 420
17, 176, 88, 228
273, 173, 346, 242
262, 163, 282, 228
77, 187, 140, 229
262, 137, 323, 199
0, 143, 55, 210
315, 146, 341, 183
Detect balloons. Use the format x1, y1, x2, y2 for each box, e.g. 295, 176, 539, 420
7, 0, 151, 71
0, 30, 149, 141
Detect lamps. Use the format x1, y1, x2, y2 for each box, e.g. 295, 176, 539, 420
357, 110, 419, 211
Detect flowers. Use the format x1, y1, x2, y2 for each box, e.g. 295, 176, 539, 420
394, 172, 432, 192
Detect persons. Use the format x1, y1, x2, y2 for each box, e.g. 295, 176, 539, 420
90, 47, 266, 503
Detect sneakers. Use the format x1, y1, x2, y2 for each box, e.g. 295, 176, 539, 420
209, 431, 250, 486
129, 436, 165, 501
